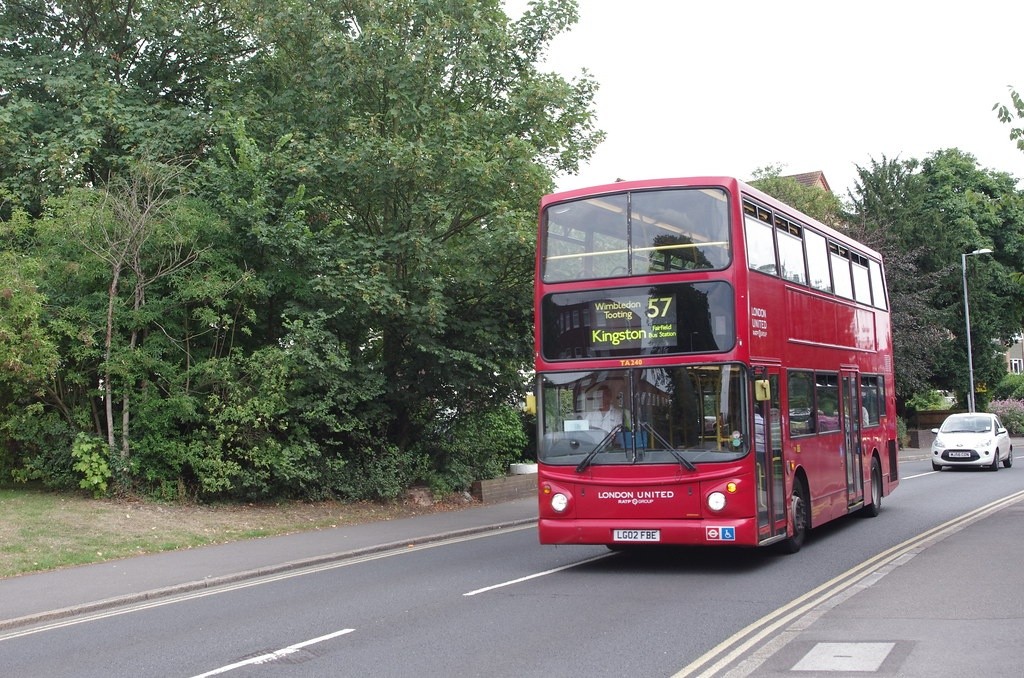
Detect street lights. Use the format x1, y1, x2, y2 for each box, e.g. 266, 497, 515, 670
960, 248, 994, 412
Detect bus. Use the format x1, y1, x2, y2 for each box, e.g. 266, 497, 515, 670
523, 174, 901, 547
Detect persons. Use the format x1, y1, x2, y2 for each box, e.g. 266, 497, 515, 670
754, 405, 767, 491
862, 406, 869, 426
585, 386, 622, 433
818, 398, 840, 431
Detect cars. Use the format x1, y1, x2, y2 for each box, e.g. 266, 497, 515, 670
930, 412, 1014, 472
789, 410, 850, 435
